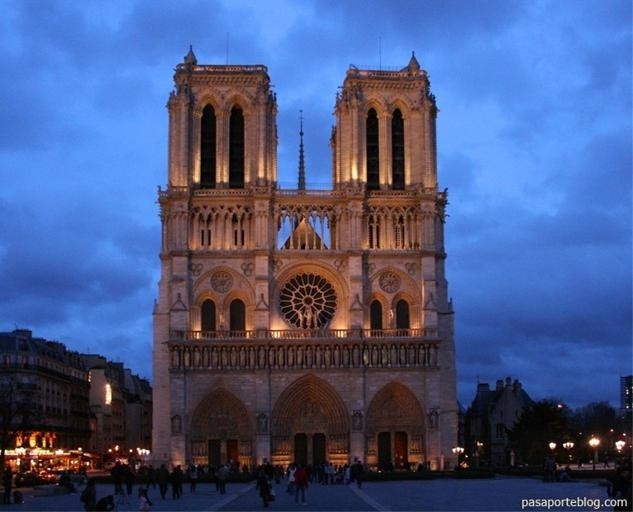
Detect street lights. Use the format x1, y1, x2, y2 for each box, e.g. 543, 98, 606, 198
588, 437, 601, 470
549, 440, 557, 470
615, 440, 626, 468
471, 441, 485, 468
562, 442, 576, 471
451, 446, 465, 472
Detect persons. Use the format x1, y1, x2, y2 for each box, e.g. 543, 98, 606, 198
81, 477, 98, 512
542, 453, 610, 483
110, 456, 364, 511
96, 494, 114, 512
1, 464, 14, 505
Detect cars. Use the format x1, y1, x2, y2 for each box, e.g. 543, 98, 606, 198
14, 469, 56, 486
